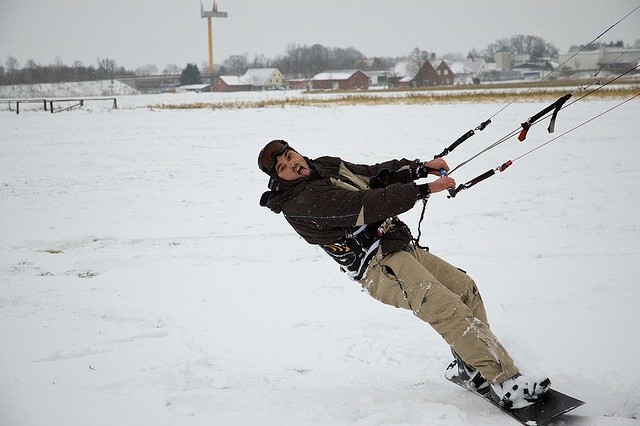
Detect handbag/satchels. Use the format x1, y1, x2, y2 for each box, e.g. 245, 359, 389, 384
380, 221, 412, 253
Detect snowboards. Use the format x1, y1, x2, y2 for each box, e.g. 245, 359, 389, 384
444, 359, 587, 426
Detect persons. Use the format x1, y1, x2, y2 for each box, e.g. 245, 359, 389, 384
258, 140, 550, 411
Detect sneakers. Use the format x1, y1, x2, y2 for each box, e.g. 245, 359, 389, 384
458, 360, 488, 395
490, 372, 551, 409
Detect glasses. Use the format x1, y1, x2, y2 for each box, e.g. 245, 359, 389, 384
259, 140, 288, 174
